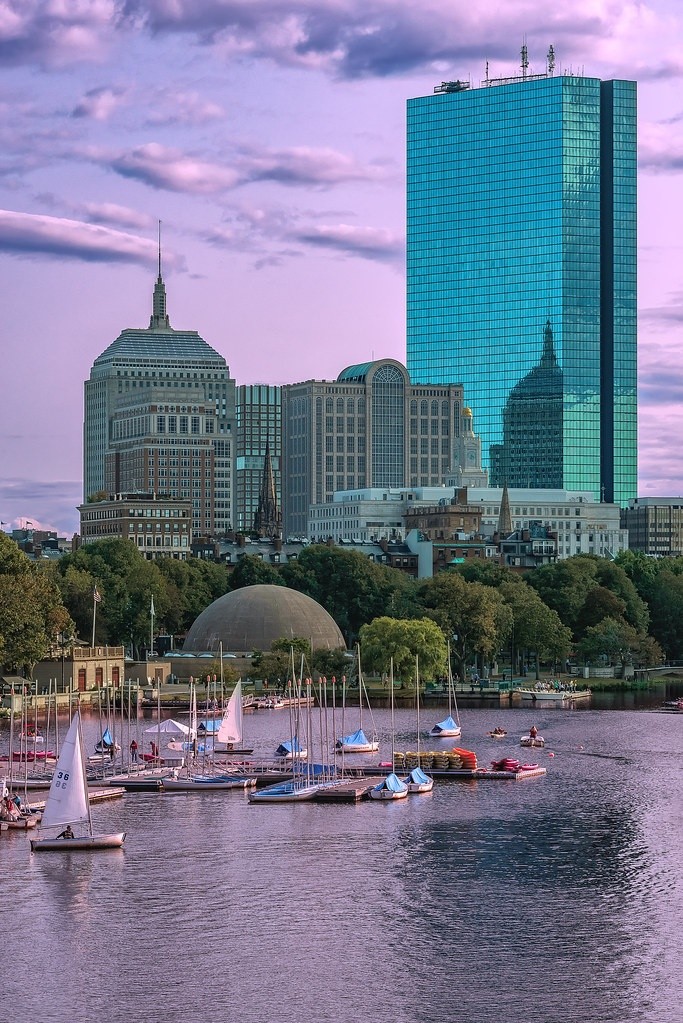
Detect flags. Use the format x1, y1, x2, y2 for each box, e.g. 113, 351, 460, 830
94, 588, 102, 602
150, 598, 155, 615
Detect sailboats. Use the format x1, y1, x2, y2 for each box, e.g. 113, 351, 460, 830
0, 641, 546, 851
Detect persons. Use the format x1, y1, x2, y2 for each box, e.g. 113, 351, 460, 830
453, 673, 458, 685
150, 741, 158, 756
633, 671, 645, 683
494, 726, 504, 735
26, 689, 32, 696
529, 726, 537, 739
28, 728, 41, 737
470, 673, 478, 684
534, 678, 577, 693
0, 792, 21, 820
130, 739, 137, 763
107, 743, 117, 759
192, 739, 199, 756
151, 678, 155, 689
523, 665, 528, 677
276, 679, 283, 689
355, 676, 359, 688
56, 825, 74, 839
227, 743, 234, 750
